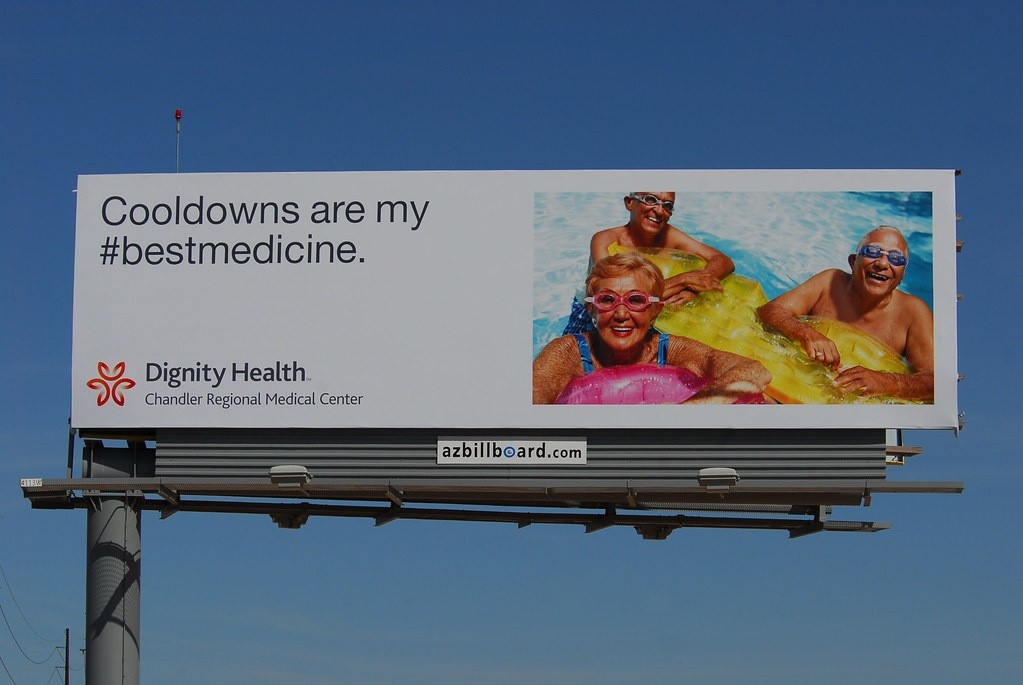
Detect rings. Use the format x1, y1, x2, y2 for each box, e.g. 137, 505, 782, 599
815, 352, 825, 356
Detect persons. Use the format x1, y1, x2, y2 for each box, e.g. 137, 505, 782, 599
756, 224, 934, 401
532, 252, 772, 404
561, 191, 735, 337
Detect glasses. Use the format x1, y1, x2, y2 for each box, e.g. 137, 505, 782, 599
584, 291, 661, 311
629, 194, 675, 216
856, 245, 907, 266
